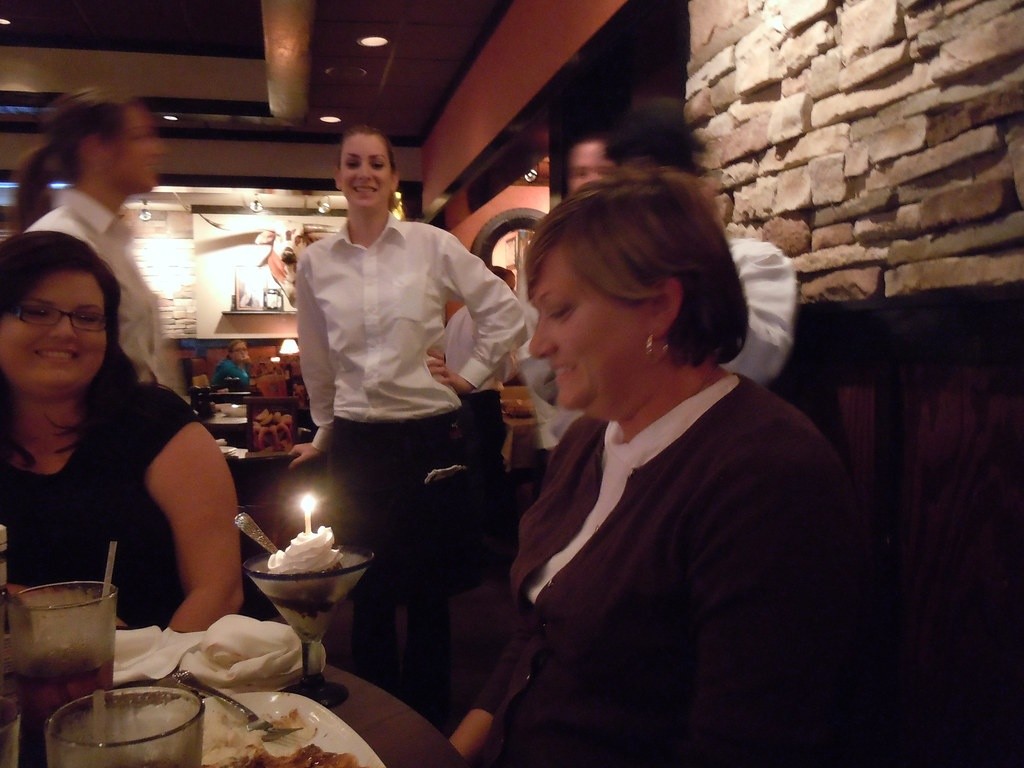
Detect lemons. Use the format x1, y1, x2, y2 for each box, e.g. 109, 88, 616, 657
254, 408, 273, 424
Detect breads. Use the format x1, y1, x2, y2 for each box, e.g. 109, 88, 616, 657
252, 422, 291, 451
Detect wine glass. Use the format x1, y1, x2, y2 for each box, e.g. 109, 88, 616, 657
242, 545, 375, 708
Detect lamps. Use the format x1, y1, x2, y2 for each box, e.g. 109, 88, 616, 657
278, 339, 300, 376
138, 194, 331, 220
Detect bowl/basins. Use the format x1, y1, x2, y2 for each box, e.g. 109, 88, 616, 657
222, 404, 247, 417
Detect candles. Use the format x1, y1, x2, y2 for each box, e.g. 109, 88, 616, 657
300, 493, 319, 532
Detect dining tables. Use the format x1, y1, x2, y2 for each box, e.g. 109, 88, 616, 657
190, 402, 249, 433
1, 620, 475, 768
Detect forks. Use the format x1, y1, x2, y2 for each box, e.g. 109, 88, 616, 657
172, 670, 303, 742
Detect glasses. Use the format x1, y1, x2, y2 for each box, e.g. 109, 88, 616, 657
0, 303, 109, 332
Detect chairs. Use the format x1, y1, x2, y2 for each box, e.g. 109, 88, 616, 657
221, 455, 325, 556
182, 357, 212, 393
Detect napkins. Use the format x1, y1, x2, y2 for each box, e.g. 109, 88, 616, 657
113, 613, 306, 693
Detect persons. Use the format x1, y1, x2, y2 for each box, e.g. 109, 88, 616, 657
446, 167, 912, 768
210, 340, 253, 393
285, 121, 531, 735
0, 89, 176, 389
0, 231, 276, 640
513, 99, 808, 496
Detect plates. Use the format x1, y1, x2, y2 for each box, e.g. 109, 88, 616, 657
200, 691, 385, 768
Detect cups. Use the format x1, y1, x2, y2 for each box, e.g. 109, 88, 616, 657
43, 687, 206, 768
6, 582, 119, 752
1, 697, 21, 768
188, 385, 212, 421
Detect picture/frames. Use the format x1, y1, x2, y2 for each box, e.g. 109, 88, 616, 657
235, 265, 267, 310
